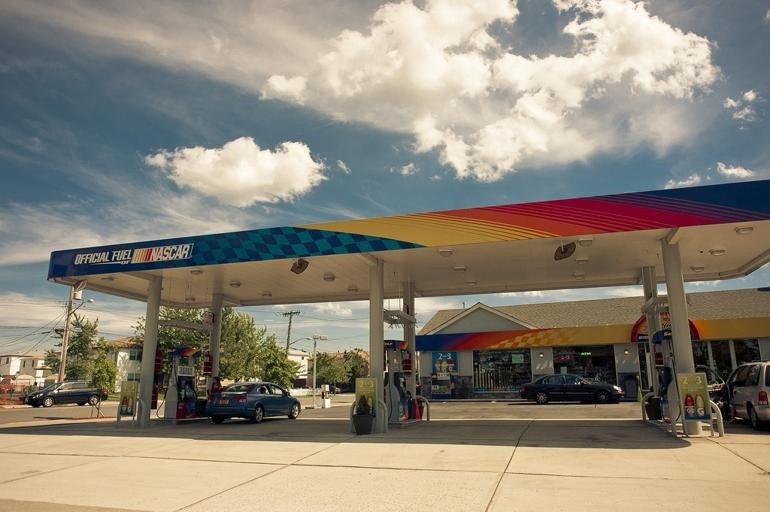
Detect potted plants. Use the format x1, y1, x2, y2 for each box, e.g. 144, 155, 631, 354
415, 385, 427, 420
644, 386, 663, 421
352, 393, 375, 435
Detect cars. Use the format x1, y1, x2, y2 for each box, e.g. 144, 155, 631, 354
328, 385, 342, 394
206, 382, 303, 421
18, 379, 111, 410
519, 372, 624, 404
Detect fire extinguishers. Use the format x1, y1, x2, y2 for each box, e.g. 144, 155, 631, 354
151, 382, 157, 410
203, 351, 213, 375
401, 348, 412, 376
154, 348, 162, 373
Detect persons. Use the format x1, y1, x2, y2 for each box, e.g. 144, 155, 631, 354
207, 376, 224, 393
259, 387, 266, 394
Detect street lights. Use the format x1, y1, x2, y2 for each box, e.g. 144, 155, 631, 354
285, 336, 314, 355
56, 299, 95, 383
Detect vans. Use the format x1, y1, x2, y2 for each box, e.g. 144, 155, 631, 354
690, 360, 770, 431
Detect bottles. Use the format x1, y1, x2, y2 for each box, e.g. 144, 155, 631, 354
367, 394, 373, 413
120, 396, 128, 415
125, 396, 134, 416
685, 393, 696, 419
695, 392, 706, 418
434, 352, 455, 373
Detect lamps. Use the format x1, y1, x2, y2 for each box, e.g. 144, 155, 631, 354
438, 247, 454, 256
228, 279, 240, 288
188, 267, 203, 276
262, 290, 273, 297
577, 237, 594, 248
574, 256, 589, 264
571, 269, 587, 280
465, 280, 477, 286
689, 265, 705, 273
345, 286, 360, 293
322, 272, 335, 281
732, 224, 755, 237
453, 265, 466, 272
707, 246, 729, 256
